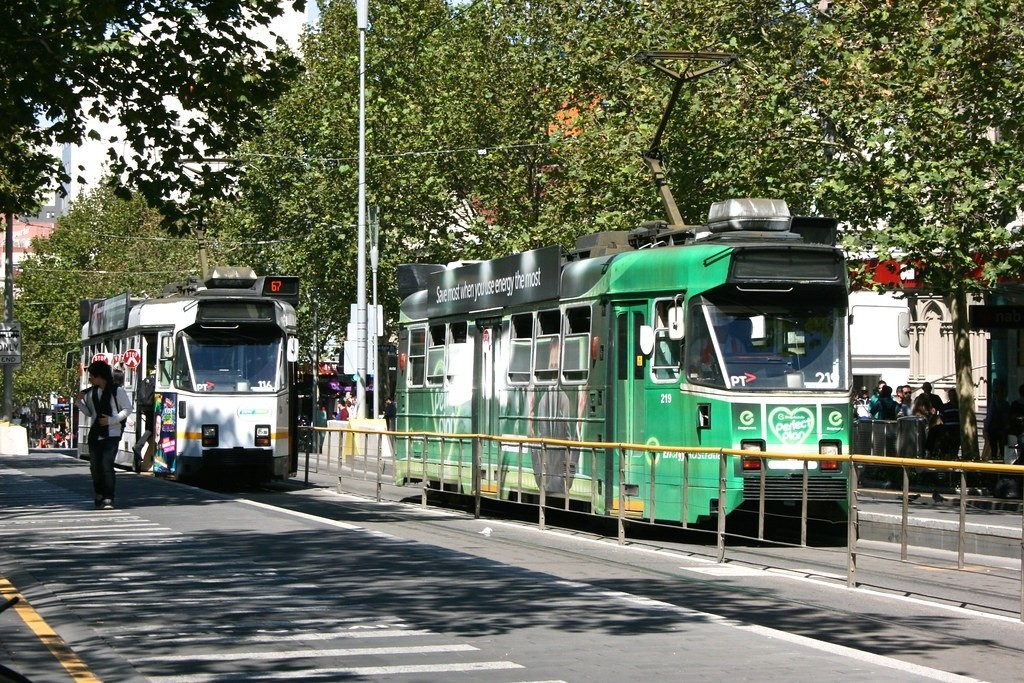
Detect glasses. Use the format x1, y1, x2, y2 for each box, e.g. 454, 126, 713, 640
903, 391, 912, 394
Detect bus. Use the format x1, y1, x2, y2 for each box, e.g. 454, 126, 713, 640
65, 221, 302, 486
389, 47, 860, 544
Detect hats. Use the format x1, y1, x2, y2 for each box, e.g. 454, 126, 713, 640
337, 399, 345, 403
860, 386, 869, 394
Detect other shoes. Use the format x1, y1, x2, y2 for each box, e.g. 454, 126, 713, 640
95, 496, 114, 509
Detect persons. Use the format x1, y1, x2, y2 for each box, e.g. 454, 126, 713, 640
383, 398, 396, 431
316, 391, 357, 446
132, 365, 156, 462
853, 378, 1024, 478
531, 336, 588, 492
689, 309, 746, 372
75, 361, 133, 509
